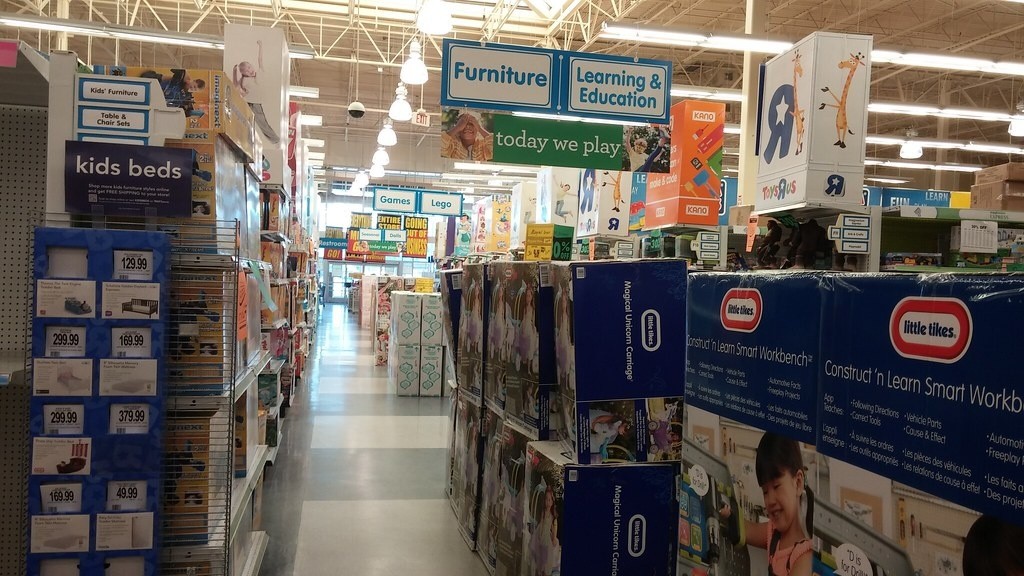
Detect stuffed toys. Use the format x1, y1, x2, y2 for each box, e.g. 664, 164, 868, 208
757, 212, 833, 268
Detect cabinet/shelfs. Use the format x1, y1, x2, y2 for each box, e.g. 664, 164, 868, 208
348, 288, 359, 314
748, 201, 1024, 274
718, 204, 1024, 270
171, 251, 271, 576
641, 223, 769, 272
260, 183, 316, 465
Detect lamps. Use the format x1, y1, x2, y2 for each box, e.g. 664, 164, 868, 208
600, 22, 1024, 183
347, 0, 455, 193
0, 11, 327, 185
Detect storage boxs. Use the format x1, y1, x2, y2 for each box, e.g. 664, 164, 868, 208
391, 293, 456, 398
755, 31, 872, 210
438, 163, 1024, 575
644, 98, 726, 229
92, 64, 319, 544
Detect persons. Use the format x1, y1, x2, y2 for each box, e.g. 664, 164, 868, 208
513, 283, 535, 375
466, 281, 481, 354
163, 69, 206, 109
231, 40, 280, 143
557, 289, 570, 389
625, 126, 671, 173
551, 172, 577, 224
441, 111, 493, 161
491, 286, 504, 361
527, 487, 558, 576
484, 440, 499, 518
465, 426, 475, 498
522, 197, 536, 225
720, 431, 815, 576
589, 409, 633, 453
